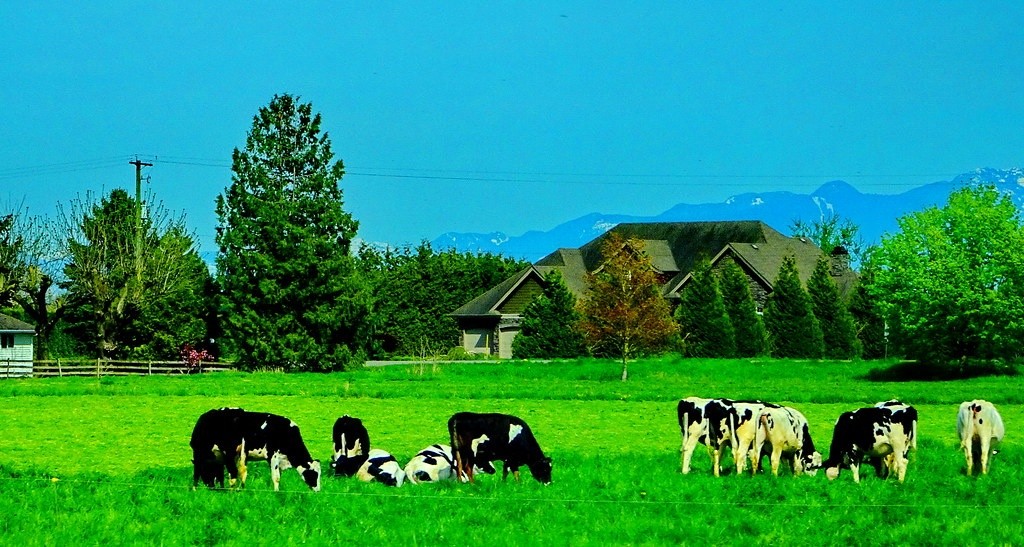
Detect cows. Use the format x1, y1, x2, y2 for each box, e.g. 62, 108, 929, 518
677, 396, 823, 478
329, 411, 554, 488
189, 406, 322, 493
822, 398, 1004, 484
